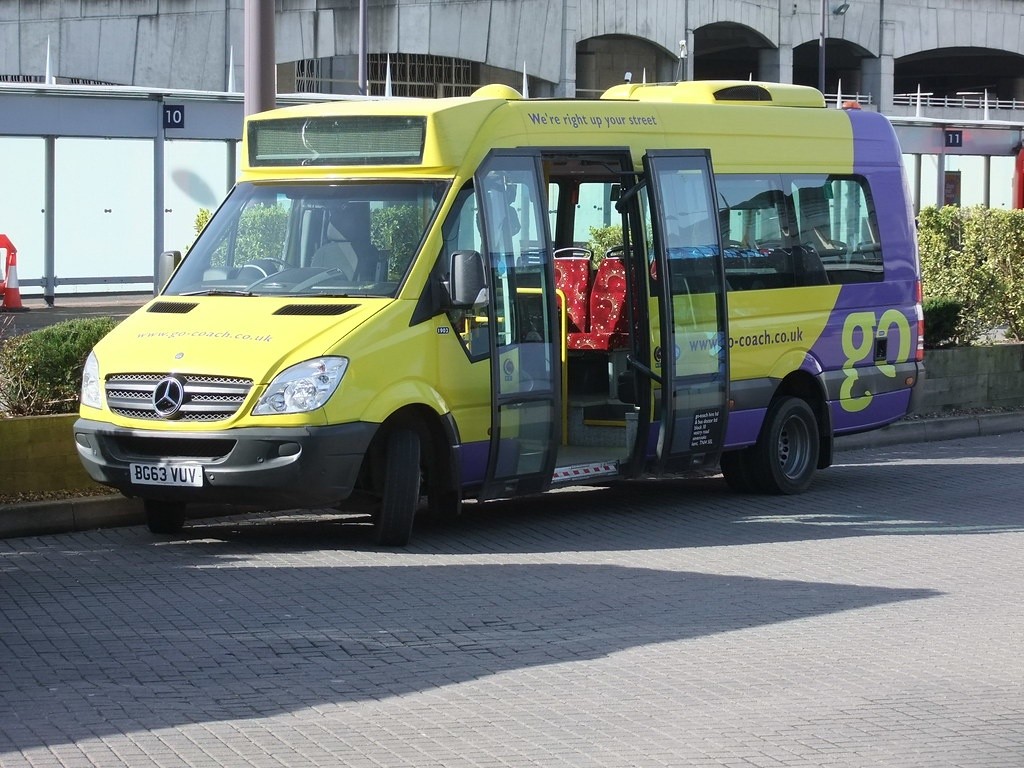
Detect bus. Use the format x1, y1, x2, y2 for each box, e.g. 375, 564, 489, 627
71, 76, 926, 548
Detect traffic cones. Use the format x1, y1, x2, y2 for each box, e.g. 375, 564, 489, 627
0, 253, 31, 312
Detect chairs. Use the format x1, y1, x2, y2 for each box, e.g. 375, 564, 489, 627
303, 211, 368, 281
549, 248, 592, 340
569, 243, 642, 401
